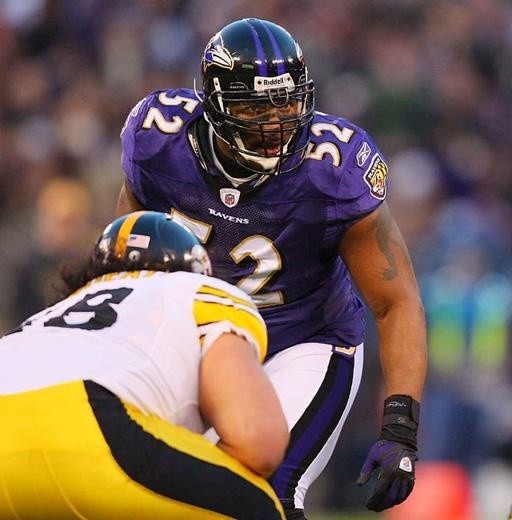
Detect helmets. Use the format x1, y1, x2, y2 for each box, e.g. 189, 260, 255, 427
91, 209, 215, 274
200, 16, 318, 176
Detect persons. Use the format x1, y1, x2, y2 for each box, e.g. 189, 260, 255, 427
1, 2, 512, 519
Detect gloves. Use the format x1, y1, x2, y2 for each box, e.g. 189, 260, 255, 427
353, 393, 423, 515
